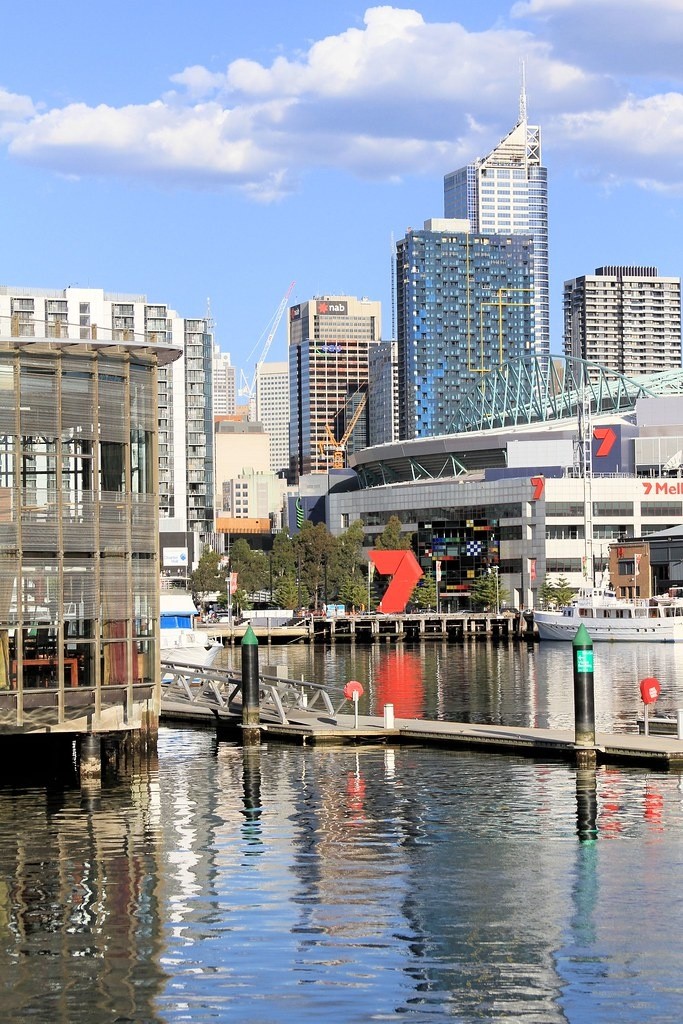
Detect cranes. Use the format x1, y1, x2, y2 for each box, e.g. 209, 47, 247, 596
202, 293, 266, 336
238, 279, 297, 422
316, 342, 396, 469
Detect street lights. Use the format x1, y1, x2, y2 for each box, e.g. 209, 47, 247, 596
224, 576, 232, 623
492, 565, 500, 616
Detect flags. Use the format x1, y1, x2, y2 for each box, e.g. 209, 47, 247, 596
370, 562, 376, 583
231, 573, 238, 594
531, 560, 536, 581
583, 557, 587, 577
437, 561, 441, 581
635, 554, 642, 575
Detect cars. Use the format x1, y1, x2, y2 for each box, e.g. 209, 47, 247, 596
418, 608, 437, 615
362, 611, 385, 616
456, 609, 474, 614
499, 607, 519, 614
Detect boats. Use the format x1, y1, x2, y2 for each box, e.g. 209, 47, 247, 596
531, 561, 683, 643
159, 589, 225, 688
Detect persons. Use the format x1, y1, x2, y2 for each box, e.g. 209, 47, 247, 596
352, 604, 355, 614
361, 604, 365, 615
321, 603, 326, 616
484, 604, 490, 613
